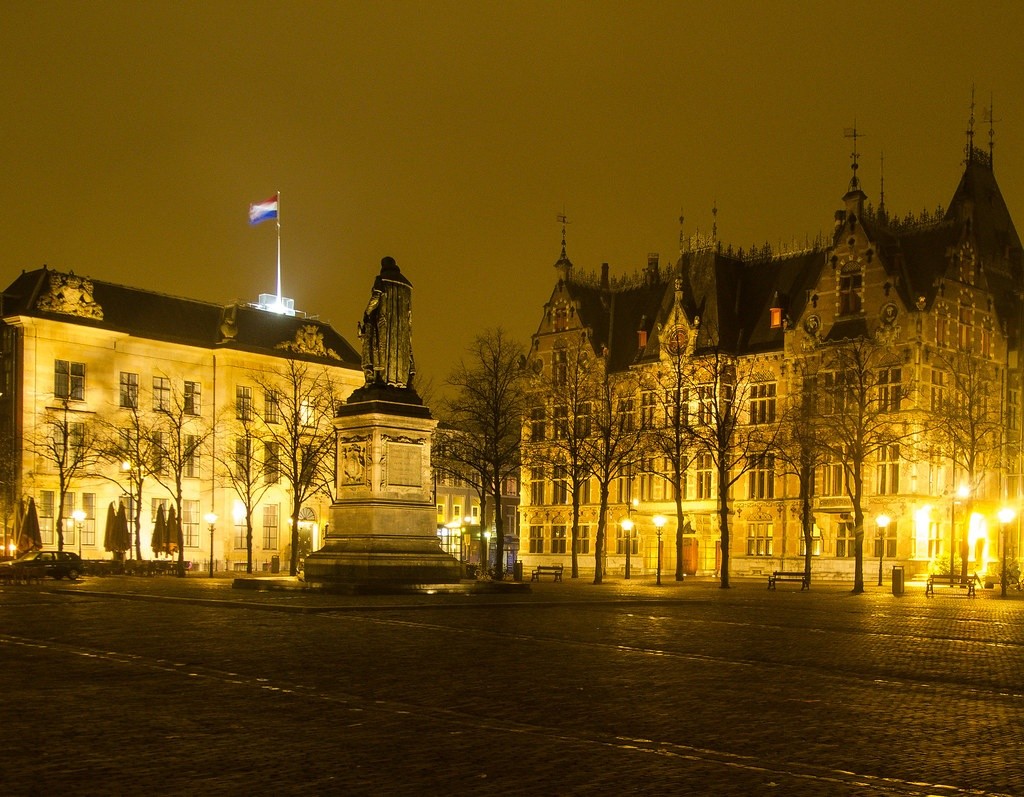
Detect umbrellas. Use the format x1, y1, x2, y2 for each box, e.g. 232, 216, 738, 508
103, 498, 130, 565
150, 502, 179, 562
11, 496, 43, 554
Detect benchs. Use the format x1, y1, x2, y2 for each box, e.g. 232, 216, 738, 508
924, 573, 977, 597
768, 571, 811, 591
530, 564, 563, 582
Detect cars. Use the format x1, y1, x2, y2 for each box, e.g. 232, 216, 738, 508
0, 551, 88, 580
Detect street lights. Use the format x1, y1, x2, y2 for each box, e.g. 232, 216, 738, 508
205, 511, 217, 578
74, 510, 86, 559
875, 513, 889, 586
653, 518, 666, 583
1000, 508, 1015, 599
620, 520, 633, 578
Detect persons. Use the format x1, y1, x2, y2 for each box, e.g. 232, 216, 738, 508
362, 257, 416, 390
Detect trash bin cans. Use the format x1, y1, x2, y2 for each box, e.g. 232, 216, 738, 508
892, 565, 905, 594
271, 555, 279, 573
513, 559, 523, 582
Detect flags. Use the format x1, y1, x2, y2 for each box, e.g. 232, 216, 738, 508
248, 193, 277, 224
